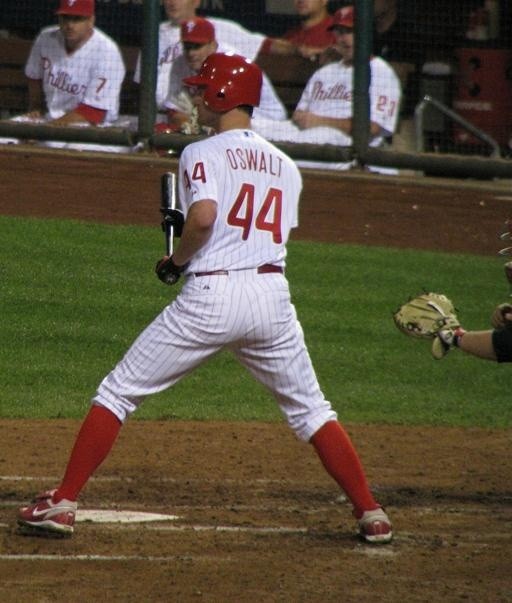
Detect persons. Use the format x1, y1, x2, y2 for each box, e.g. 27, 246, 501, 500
248, 6, 401, 177
16, 52, 395, 542
436, 300, 511, 364
274, 0, 343, 58
20, 0, 126, 128
371, 1, 427, 177
176, 17, 288, 140
131, 0, 322, 129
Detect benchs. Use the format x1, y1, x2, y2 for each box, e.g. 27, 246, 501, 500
1, 37, 453, 180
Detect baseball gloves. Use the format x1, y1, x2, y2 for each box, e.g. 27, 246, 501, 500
395, 293, 461, 357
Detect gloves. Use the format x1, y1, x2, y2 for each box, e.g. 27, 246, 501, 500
154, 253, 193, 286
160, 208, 185, 240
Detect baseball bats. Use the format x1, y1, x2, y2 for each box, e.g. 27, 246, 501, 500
161, 171, 180, 284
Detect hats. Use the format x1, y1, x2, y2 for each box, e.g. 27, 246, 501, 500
323, 6, 354, 33
179, 17, 215, 45
52, 1, 96, 19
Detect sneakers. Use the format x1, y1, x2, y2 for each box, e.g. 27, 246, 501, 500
351, 504, 394, 546
15, 488, 76, 537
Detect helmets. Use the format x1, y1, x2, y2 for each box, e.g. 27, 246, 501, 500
181, 51, 264, 115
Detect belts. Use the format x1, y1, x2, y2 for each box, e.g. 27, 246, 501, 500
196, 263, 283, 276
